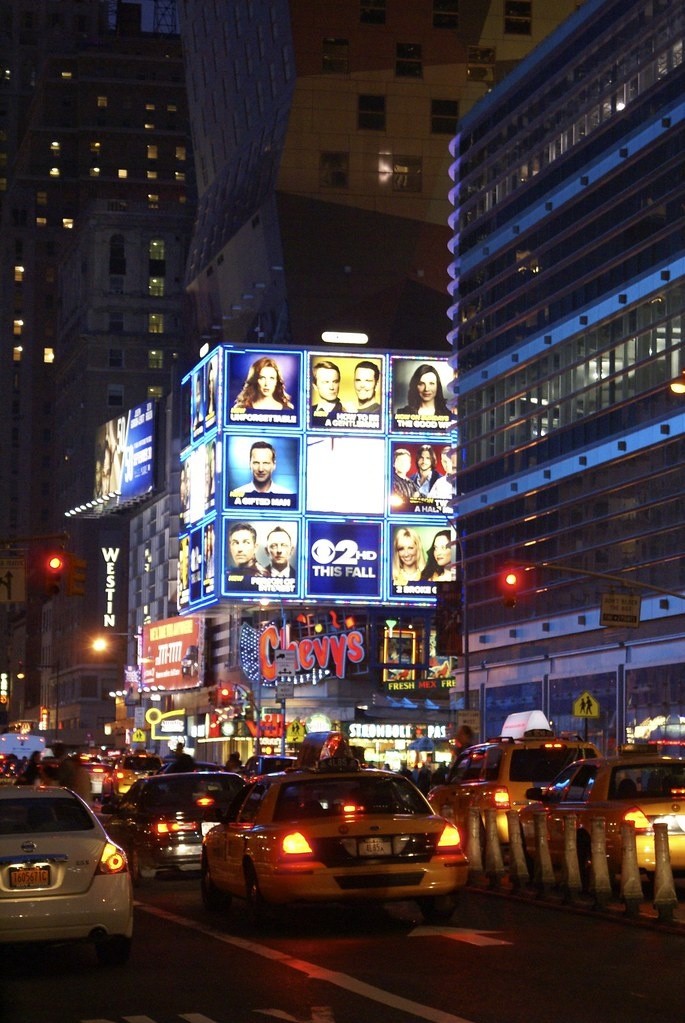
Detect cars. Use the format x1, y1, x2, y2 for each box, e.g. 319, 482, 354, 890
113, 754, 164, 802
244, 755, 298, 780
519, 752, 685, 891
102, 772, 254, 885
0, 785, 134, 967
81, 762, 111, 800
202, 770, 468, 921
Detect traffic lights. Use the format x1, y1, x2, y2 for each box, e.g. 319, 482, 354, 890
44, 550, 64, 597
66, 552, 87, 595
220, 684, 228, 700
504, 568, 515, 608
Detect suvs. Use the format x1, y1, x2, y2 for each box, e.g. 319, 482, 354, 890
426, 735, 603, 860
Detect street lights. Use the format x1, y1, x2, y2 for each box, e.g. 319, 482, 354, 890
393, 494, 468, 712
17, 661, 58, 739
96, 630, 142, 709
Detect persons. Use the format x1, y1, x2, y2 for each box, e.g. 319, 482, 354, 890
233, 358, 295, 409
230, 442, 290, 493
165, 743, 192, 772
394, 528, 451, 581
95, 421, 120, 501
179, 361, 213, 597
230, 523, 296, 577
14, 751, 91, 806
314, 361, 380, 413
396, 365, 453, 417
367, 760, 449, 792
394, 445, 454, 498
227, 754, 243, 772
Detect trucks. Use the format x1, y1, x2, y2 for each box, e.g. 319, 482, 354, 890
0, 732, 47, 761
